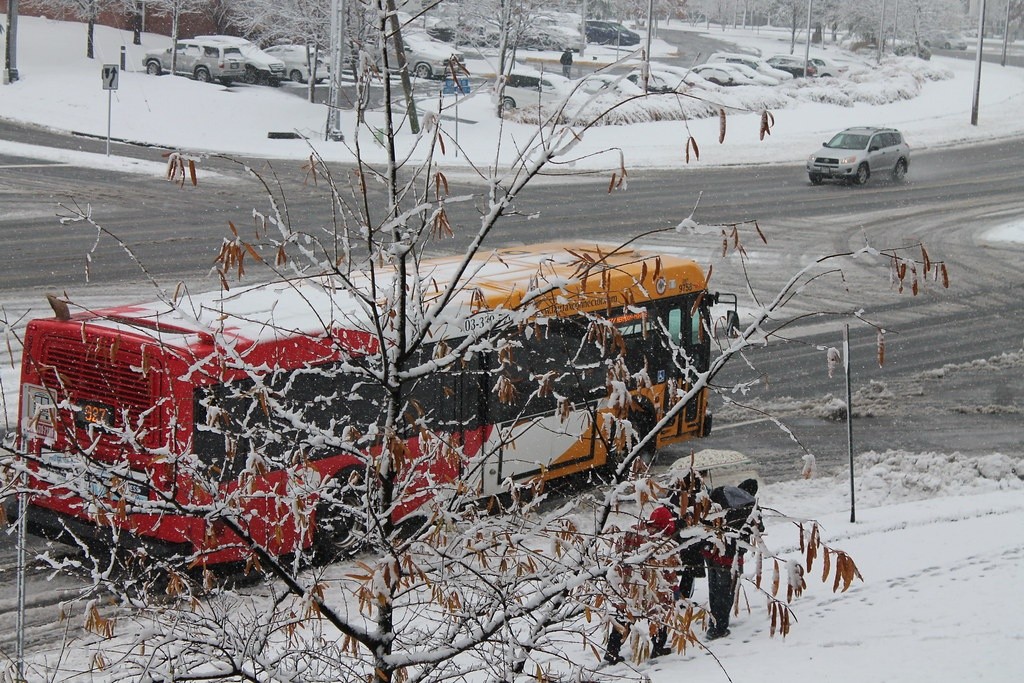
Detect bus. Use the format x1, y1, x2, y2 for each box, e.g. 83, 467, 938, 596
7, 238, 742, 580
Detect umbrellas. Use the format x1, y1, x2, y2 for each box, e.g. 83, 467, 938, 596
672, 449, 750, 490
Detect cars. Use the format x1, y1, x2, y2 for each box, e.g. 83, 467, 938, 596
921, 29, 966, 50
492, 70, 593, 123
808, 56, 849, 79
351, 10, 585, 79
572, 74, 650, 102
691, 52, 793, 88
626, 71, 691, 94
663, 66, 726, 94
576, 22, 640, 44
259, 44, 332, 84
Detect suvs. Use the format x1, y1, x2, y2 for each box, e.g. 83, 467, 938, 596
805, 127, 912, 185
142, 38, 247, 84
196, 35, 286, 88
766, 55, 818, 78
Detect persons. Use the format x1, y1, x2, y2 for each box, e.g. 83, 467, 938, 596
697, 479, 765, 640
603, 507, 681, 664
560, 47, 573, 79
662, 470, 707, 609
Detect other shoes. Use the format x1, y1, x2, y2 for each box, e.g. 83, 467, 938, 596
650, 638, 671, 658
603, 646, 625, 664
707, 628, 731, 641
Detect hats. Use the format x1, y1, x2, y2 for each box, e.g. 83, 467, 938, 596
651, 507, 675, 537
